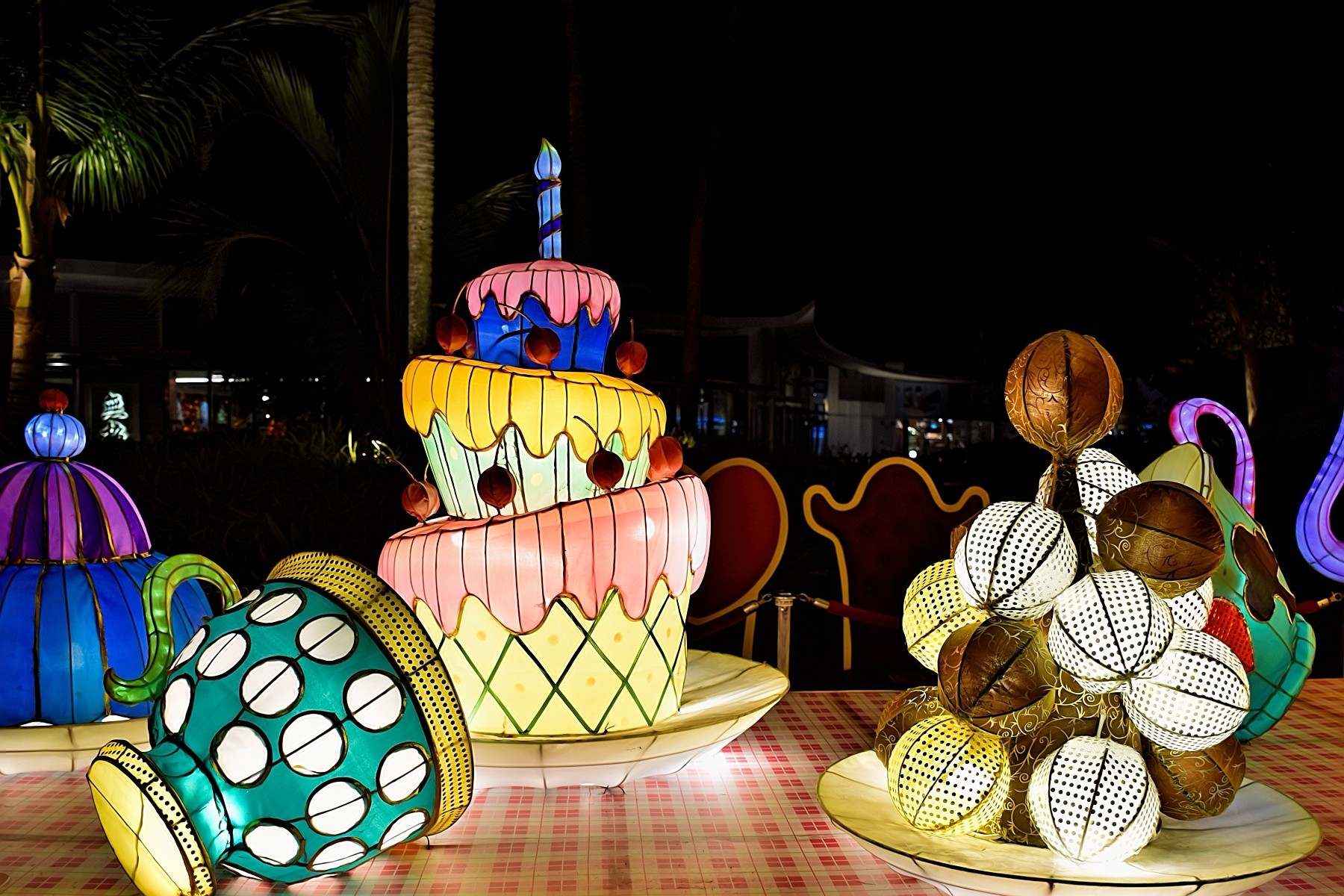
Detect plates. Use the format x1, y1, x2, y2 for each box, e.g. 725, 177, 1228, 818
470, 649, 790, 788
817, 749, 1324, 895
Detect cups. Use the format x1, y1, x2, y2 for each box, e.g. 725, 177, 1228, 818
1137, 397, 1318, 743
88, 552, 473, 895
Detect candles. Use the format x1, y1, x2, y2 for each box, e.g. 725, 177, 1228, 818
535, 137, 562, 260
